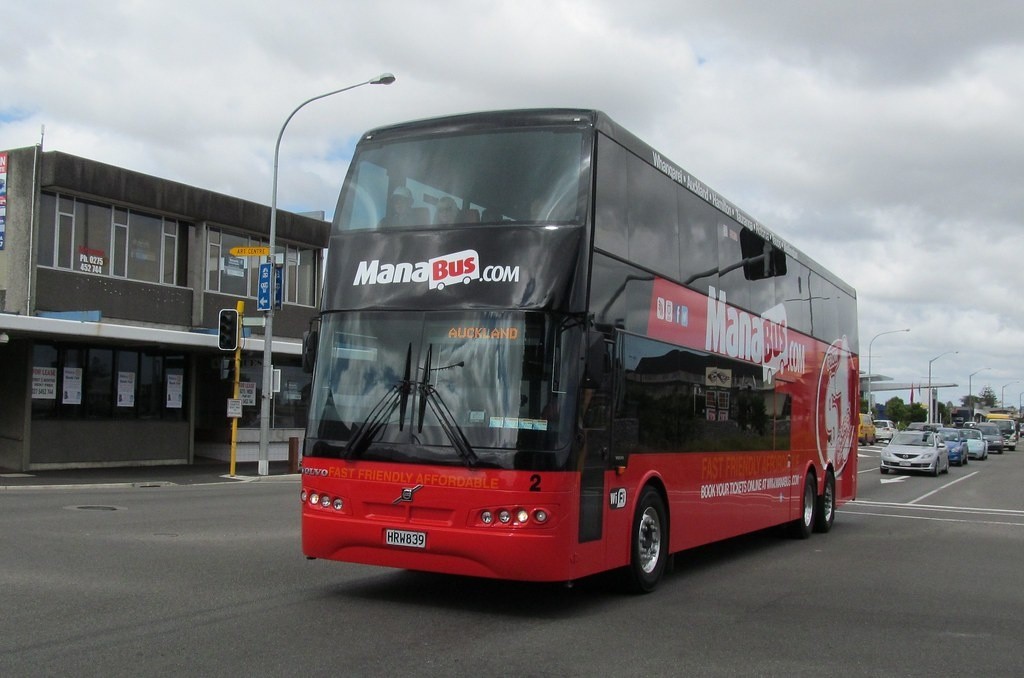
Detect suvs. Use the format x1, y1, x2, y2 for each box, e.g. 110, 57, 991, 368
908, 422, 943, 432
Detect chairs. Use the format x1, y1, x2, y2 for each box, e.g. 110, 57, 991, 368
468, 209, 480, 221
410, 207, 430, 225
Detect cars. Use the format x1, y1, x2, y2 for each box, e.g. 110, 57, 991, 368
873, 419, 899, 443
879, 426, 949, 477
1019, 423, 1024, 437
936, 428, 968, 467
961, 429, 988, 460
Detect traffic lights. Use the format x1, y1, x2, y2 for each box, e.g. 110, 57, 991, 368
216, 309, 239, 351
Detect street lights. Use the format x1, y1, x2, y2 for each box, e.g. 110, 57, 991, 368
929, 351, 959, 424
1001, 380, 1020, 414
257, 74, 398, 475
867, 329, 910, 411
969, 366, 990, 421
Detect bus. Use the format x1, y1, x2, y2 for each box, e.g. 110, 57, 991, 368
986, 414, 1010, 421
299, 110, 859, 594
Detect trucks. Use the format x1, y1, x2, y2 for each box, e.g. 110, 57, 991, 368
987, 418, 1017, 450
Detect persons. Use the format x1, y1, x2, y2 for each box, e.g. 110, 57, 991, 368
482, 208, 503, 222
547, 346, 593, 470
377, 186, 423, 226
434, 196, 463, 224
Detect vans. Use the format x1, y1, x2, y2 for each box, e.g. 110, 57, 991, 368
858, 412, 876, 445
964, 422, 978, 428
974, 422, 1003, 454
